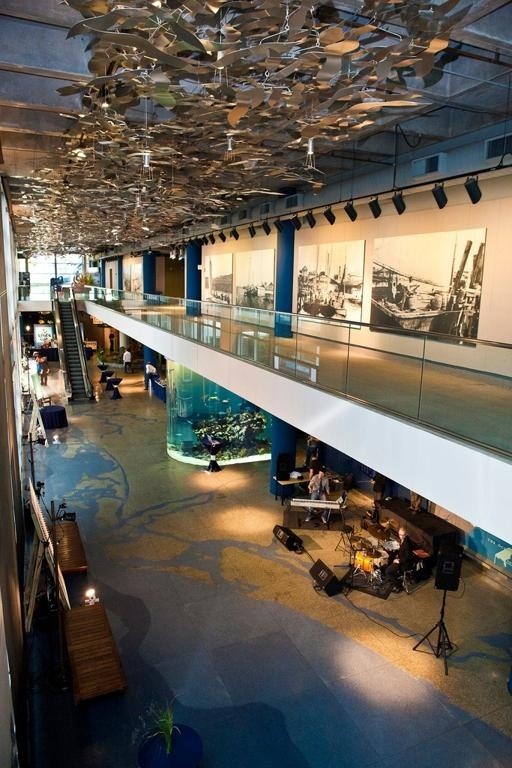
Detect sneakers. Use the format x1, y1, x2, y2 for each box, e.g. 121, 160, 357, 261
321, 516, 326, 523
304, 516, 311, 521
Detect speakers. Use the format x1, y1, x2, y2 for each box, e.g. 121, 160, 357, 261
273, 525, 303, 551
434, 545, 464, 591
276, 453, 290, 480
309, 559, 344, 596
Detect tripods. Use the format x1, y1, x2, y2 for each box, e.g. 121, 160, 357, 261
341, 551, 369, 596
412, 590, 453, 675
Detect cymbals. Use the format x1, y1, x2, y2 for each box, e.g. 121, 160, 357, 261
381, 517, 400, 530
369, 525, 391, 540
362, 546, 382, 558
349, 536, 373, 548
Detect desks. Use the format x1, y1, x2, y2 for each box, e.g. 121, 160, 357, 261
374, 497, 460, 557
98, 364, 122, 400
151, 379, 166, 402
272, 473, 311, 506
39, 406, 68, 430
31, 348, 58, 361
137, 724, 203, 768
290, 498, 340, 529
202, 438, 223, 472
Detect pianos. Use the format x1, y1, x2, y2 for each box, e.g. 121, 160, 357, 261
291, 498, 341, 510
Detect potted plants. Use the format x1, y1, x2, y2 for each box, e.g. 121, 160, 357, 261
143, 701, 182, 755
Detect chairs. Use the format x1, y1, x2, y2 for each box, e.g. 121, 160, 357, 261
324, 491, 348, 525
402, 557, 421, 593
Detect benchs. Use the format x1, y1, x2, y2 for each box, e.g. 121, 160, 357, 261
62, 602, 128, 700
47, 520, 89, 593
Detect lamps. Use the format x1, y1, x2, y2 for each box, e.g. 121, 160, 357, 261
323, 206, 335, 225
432, 183, 447, 209
305, 137, 315, 154
228, 139, 232, 151
464, 176, 482, 204
147, 214, 302, 251
392, 191, 405, 215
368, 196, 382, 218
343, 200, 357, 221
305, 210, 316, 228
140, 98, 152, 168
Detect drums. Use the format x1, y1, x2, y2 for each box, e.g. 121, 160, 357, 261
374, 550, 388, 566
353, 552, 374, 572
383, 541, 399, 557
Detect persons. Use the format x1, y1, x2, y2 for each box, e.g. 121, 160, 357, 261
304, 467, 331, 525
384, 526, 415, 594
109, 330, 115, 352
123, 348, 134, 374
33, 349, 51, 386
143, 361, 159, 390
372, 471, 386, 505
306, 436, 321, 467
